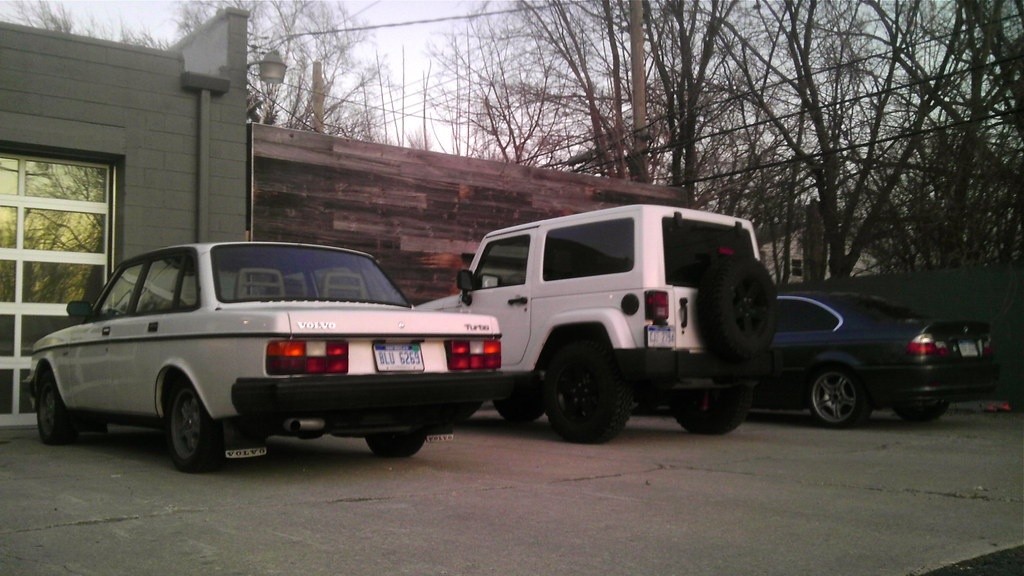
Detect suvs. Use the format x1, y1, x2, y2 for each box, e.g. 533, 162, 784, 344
414, 204, 778, 444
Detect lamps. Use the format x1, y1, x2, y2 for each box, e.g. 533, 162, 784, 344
243, 48, 290, 86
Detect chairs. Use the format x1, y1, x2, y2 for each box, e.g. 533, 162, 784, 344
319, 272, 369, 302
239, 265, 285, 299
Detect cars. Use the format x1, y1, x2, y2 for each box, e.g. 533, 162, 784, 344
629, 288, 1000, 429
31, 241, 503, 473
